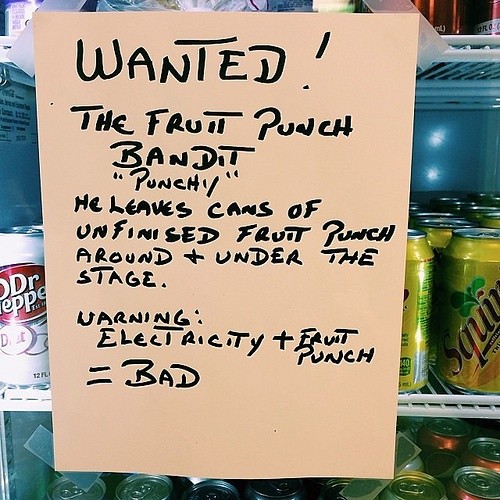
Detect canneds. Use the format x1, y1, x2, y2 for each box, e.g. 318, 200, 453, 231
4, 0, 500, 49
46, 416, 499, 500
396, 192, 500, 395
0, 223, 50, 386
